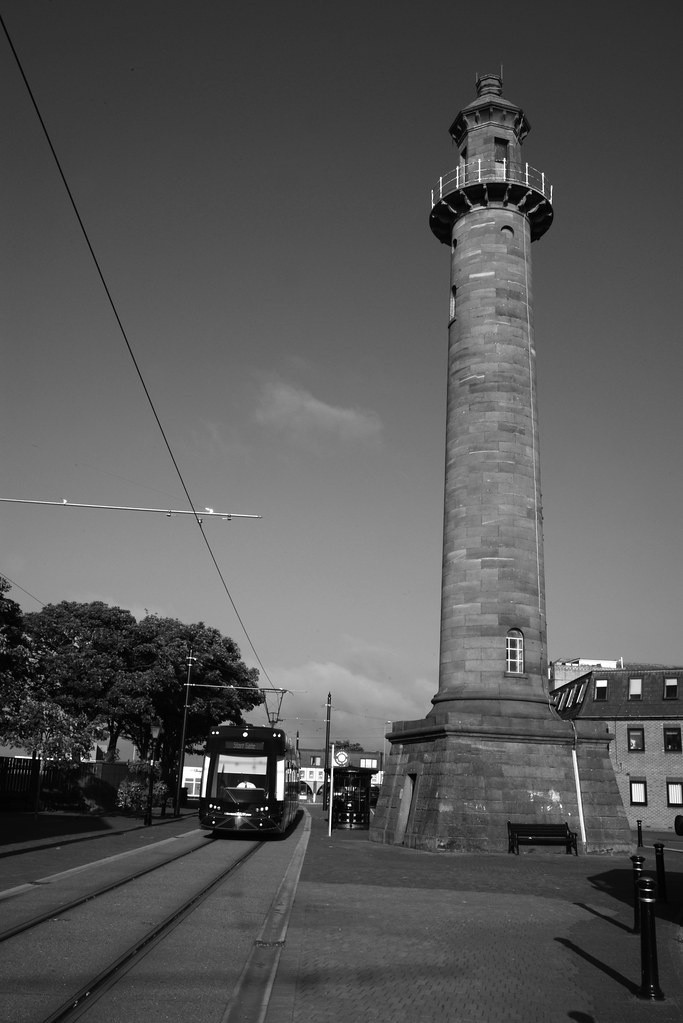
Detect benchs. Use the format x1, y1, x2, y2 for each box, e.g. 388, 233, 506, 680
506, 820, 578, 857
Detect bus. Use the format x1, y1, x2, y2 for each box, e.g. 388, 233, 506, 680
198, 687, 302, 833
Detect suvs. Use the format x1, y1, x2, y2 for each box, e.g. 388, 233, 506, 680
369, 786, 379, 807
